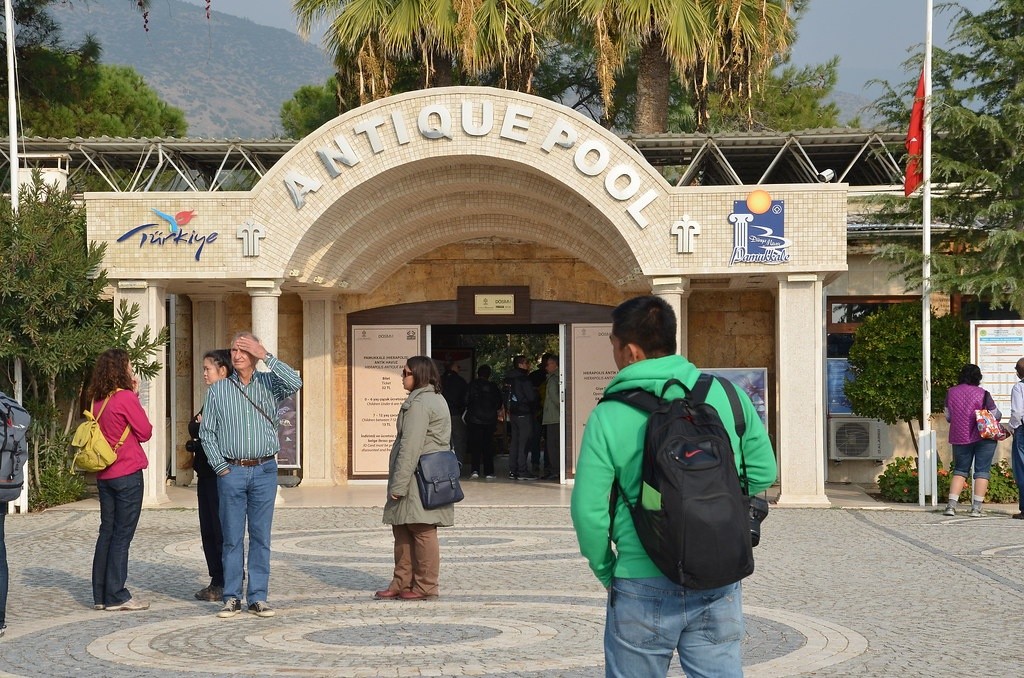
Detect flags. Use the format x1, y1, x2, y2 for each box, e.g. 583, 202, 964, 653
905, 62, 926, 198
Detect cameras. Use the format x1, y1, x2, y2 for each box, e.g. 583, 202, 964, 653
749, 495, 769, 547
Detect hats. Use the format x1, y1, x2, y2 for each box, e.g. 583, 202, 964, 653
537, 352, 558, 369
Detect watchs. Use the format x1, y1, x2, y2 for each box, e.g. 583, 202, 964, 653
263, 353, 272, 362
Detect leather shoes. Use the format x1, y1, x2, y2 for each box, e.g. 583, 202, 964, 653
372, 589, 400, 601
399, 590, 438, 602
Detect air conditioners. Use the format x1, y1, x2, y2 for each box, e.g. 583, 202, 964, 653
828, 417, 893, 464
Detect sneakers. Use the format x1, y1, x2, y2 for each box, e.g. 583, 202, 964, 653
247, 600, 275, 617
219, 598, 241, 618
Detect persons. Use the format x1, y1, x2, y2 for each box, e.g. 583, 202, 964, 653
1008, 357, 1024, 519
0, 501, 9, 637
442, 352, 560, 481
374, 356, 451, 601
943, 363, 1003, 517
86, 348, 152, 612
199, 332, 303, 617
187, 349, 246, 601
570, 296, 779, 678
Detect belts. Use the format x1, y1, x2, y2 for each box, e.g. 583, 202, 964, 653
230, 456, 274, 468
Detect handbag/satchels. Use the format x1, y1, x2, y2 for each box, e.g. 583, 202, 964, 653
462, 404, 470, 427
973, 391, 1011, 441
414, 451, 464, 509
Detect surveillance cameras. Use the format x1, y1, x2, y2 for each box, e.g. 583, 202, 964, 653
817, 169, 834, 183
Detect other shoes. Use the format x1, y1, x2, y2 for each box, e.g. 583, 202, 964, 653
943, 504, 957, 515
970, 504, 988, 517
94, 603, 105, 609
0, 628, 4, 638
517, 474, 538, 480
466, 470, 479, 479
105, 597, 150, 611
1012, 512, 1024, 520
194, 584, 223, 601
485, 473, 497, 479
509, 472, 520, 479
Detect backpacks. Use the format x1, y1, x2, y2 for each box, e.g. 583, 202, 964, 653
501, 376, 521, 411
472, 381, 500, 422
596, 371, 755, 589
67, 387, 132, 475
0, 391, 32, 501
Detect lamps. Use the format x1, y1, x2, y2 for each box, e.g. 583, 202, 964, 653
289, 270, 351, 290
618, 268, 642, 286
817, 169, 838, 183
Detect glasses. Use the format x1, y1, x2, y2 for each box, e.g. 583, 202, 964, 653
403, 369, 413, 378
